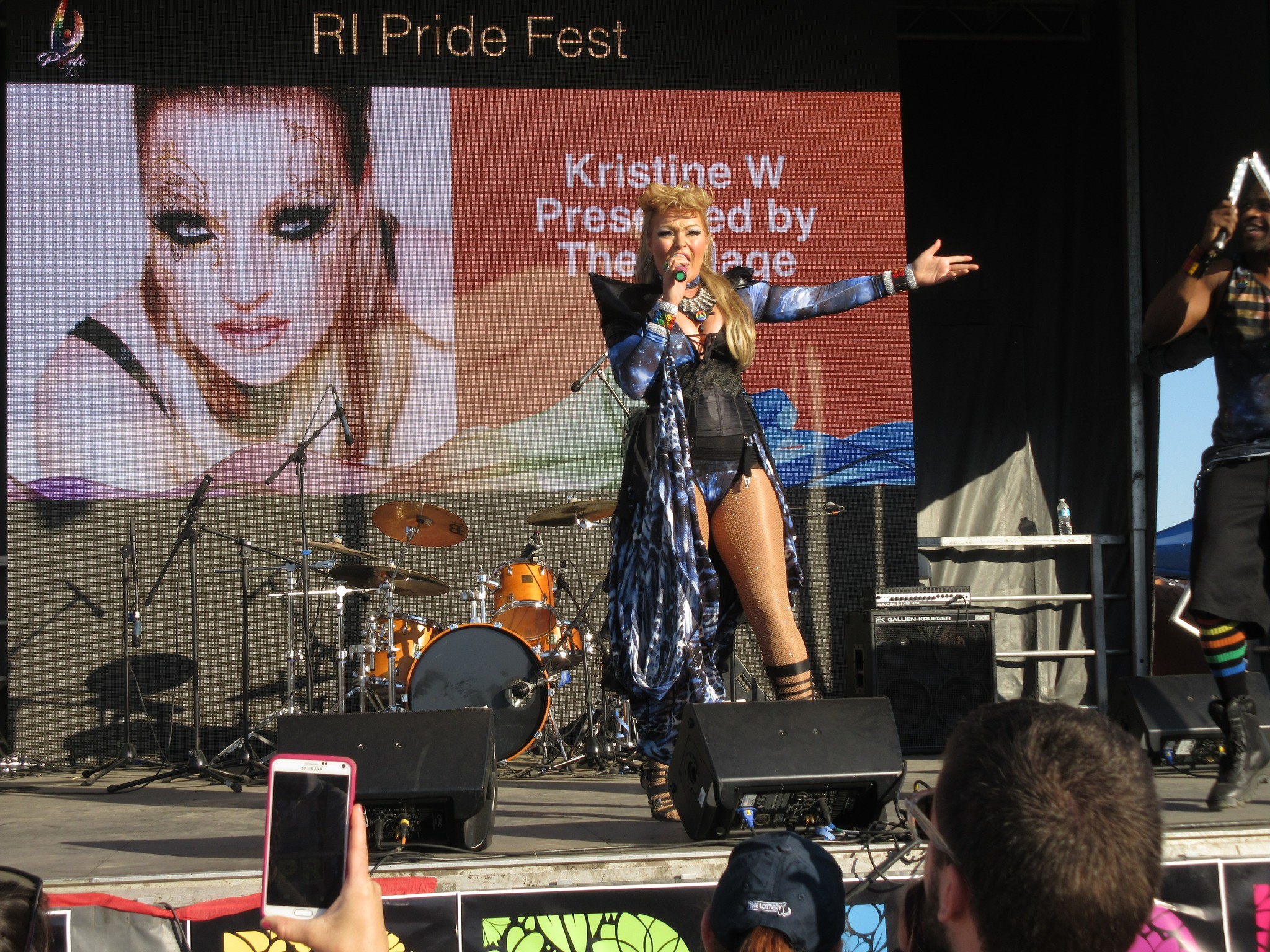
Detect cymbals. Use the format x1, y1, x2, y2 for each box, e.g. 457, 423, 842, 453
372, 501, 468, 548
287, 539, 381, 561
329, 564, 451, 597
526, 498, 618, 527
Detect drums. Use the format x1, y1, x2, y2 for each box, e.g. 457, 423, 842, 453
352, 611, 451, 697
529, 620, 586, 668
491, 558, 559, 641
400, 621, 555, 768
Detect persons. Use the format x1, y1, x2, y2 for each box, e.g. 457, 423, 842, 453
584, 181, 981, 824
30, 82, 452, 492
925, 696, 1167, 952
700, 829, 846, 952
1139, 166, 1270, 812
259, 803, 391, 952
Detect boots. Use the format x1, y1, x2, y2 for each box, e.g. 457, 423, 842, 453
1208, 697, 1270, 809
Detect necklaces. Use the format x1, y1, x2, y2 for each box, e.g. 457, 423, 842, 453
659, 271, 717, 333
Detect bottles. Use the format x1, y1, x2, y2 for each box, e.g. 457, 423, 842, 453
1056, 498, 1074, 535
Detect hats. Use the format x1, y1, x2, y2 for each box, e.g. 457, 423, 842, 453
710, 830, 845, 952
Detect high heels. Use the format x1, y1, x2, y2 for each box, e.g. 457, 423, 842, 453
639, 766, 682, 822
763, 656, 817, 701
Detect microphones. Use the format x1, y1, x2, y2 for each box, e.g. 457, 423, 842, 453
554, 561, 566, 606
519, 531, 539, 558
674, 255, 687, 284
571, 351, 609, 393
513, 674, 563, 699
132, 613, 142, 647
179, 474, 215, 523
351, 585, 371, 601
1213, 197, 1231, 252
331, 384, 354, 446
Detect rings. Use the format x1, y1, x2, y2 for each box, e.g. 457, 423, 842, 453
662, 260, 671, 273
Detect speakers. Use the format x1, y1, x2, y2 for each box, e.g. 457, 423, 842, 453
668, 695, 906, 841
277, 710, 498, 850
1122, 674, 1270, 768
853, 588, 995, 756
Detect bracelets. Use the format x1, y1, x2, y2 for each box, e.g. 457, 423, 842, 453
644, 299, 679, 339
1180, 239, 1216, 279
882, 263, 919, 296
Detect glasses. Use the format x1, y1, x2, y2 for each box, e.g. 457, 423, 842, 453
904, 788, 967, 880
0, 865, 44, 952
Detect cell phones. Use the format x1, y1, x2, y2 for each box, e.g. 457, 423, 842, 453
260, 756, 358, 924
